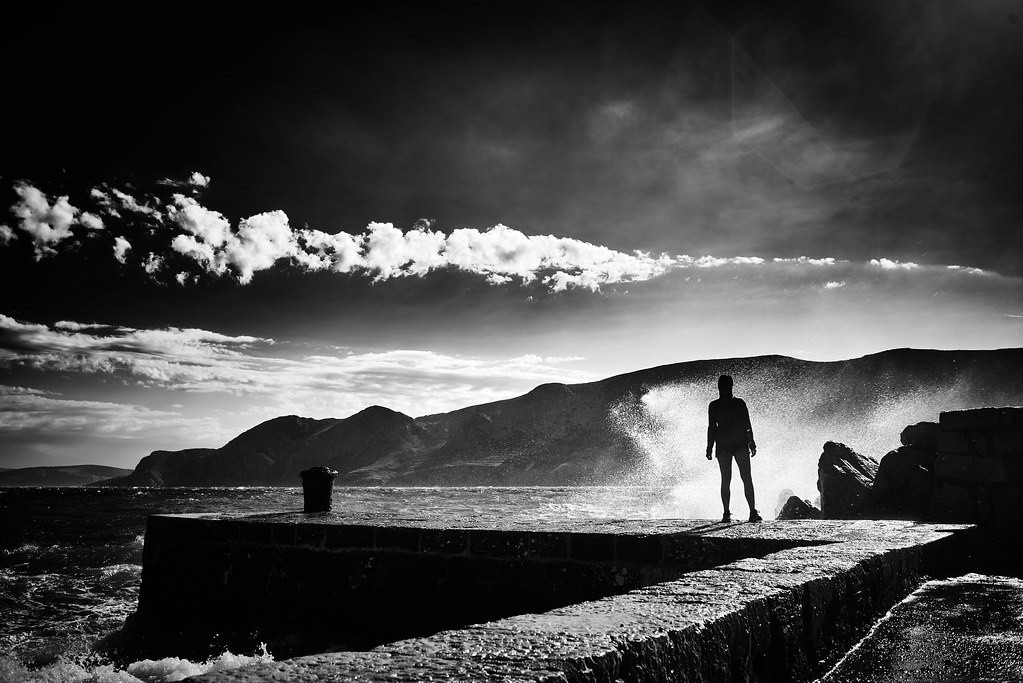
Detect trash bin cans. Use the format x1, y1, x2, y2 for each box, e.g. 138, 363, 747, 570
300, 466, 338, 512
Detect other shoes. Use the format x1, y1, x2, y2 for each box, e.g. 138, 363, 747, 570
722, 513, 731, 523
749, 509, 763, 522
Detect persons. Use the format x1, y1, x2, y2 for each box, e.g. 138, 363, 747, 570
706, 375, 763, 523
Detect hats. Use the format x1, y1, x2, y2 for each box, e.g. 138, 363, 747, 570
718, 375, 733, 386
